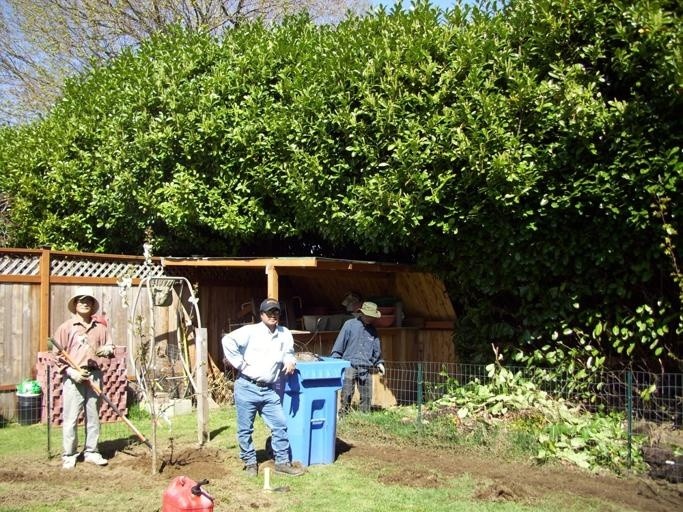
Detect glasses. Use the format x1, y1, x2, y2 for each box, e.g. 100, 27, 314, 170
264, 310, 280, 315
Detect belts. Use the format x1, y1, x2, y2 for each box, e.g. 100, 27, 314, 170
81, 366, 98, 372
240, 373, 274, 387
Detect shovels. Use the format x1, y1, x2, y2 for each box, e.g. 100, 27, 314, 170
49, 338, 173, 466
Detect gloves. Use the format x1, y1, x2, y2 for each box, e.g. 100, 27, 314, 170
377, 363, 386, 377
65, 366, 90, 384
96, 346, 113, 358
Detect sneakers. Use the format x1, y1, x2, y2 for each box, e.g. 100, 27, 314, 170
84, 452, 108, 465
62, 456, 77, 470
246, 464, 257, 476
275, 462, 304, 476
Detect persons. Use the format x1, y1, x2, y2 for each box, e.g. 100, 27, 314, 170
328, 302, 385, 419
51, 286, 113, 468
221, 298, 304, 479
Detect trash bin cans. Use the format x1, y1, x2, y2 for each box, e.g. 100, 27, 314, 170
266, 356, 351, 466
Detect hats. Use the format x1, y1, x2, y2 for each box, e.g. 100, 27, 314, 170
356, 301, 382, 319
67, 286, 99, 316
260, 298, 280, 312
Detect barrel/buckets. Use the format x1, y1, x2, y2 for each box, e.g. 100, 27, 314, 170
162, 476, 214, 512
18, 395, 41, 424
